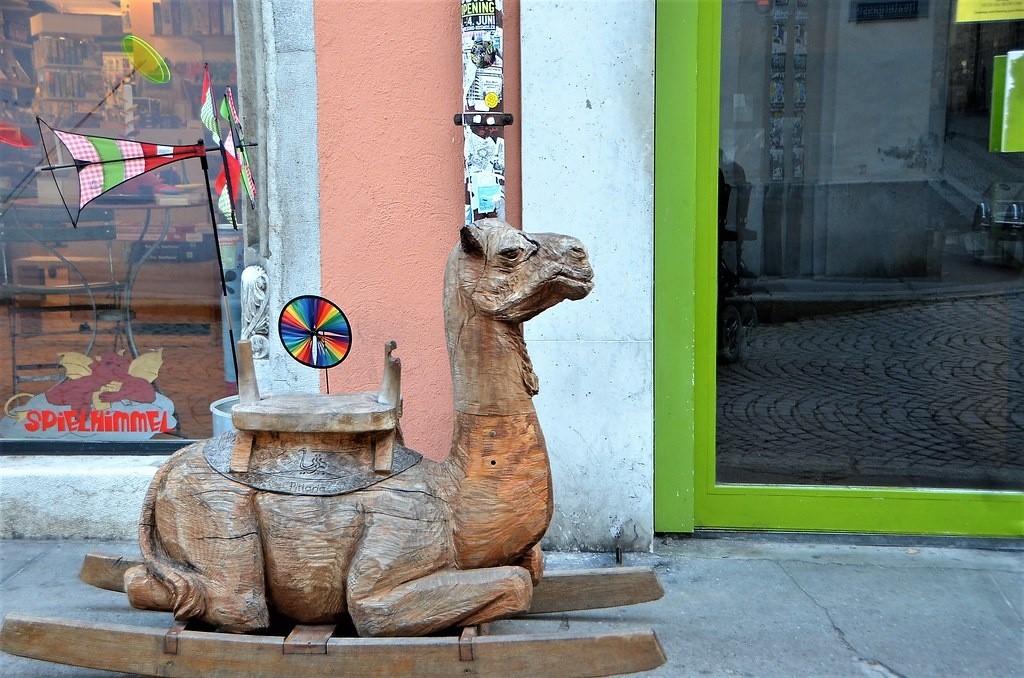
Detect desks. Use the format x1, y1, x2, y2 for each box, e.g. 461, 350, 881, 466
9, 195, 212, 440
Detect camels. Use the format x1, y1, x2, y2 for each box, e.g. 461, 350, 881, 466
129, 218, 596, 633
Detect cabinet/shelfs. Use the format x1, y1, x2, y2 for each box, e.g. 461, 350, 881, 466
30, 12, 103, 128
0, 0, 37, 198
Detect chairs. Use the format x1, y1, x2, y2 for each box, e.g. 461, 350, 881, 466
1, 206, 129, 409
